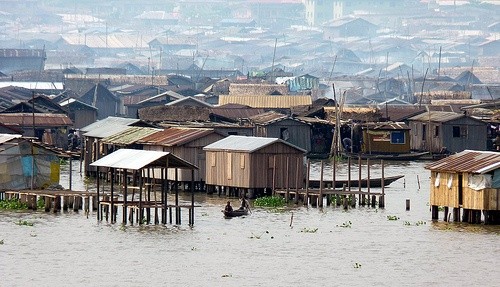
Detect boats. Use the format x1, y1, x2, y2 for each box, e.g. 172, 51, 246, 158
221, 206, 247, 218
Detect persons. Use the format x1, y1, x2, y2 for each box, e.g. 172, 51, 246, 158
239, 193, 248, 211
225, 200, 234, 212
